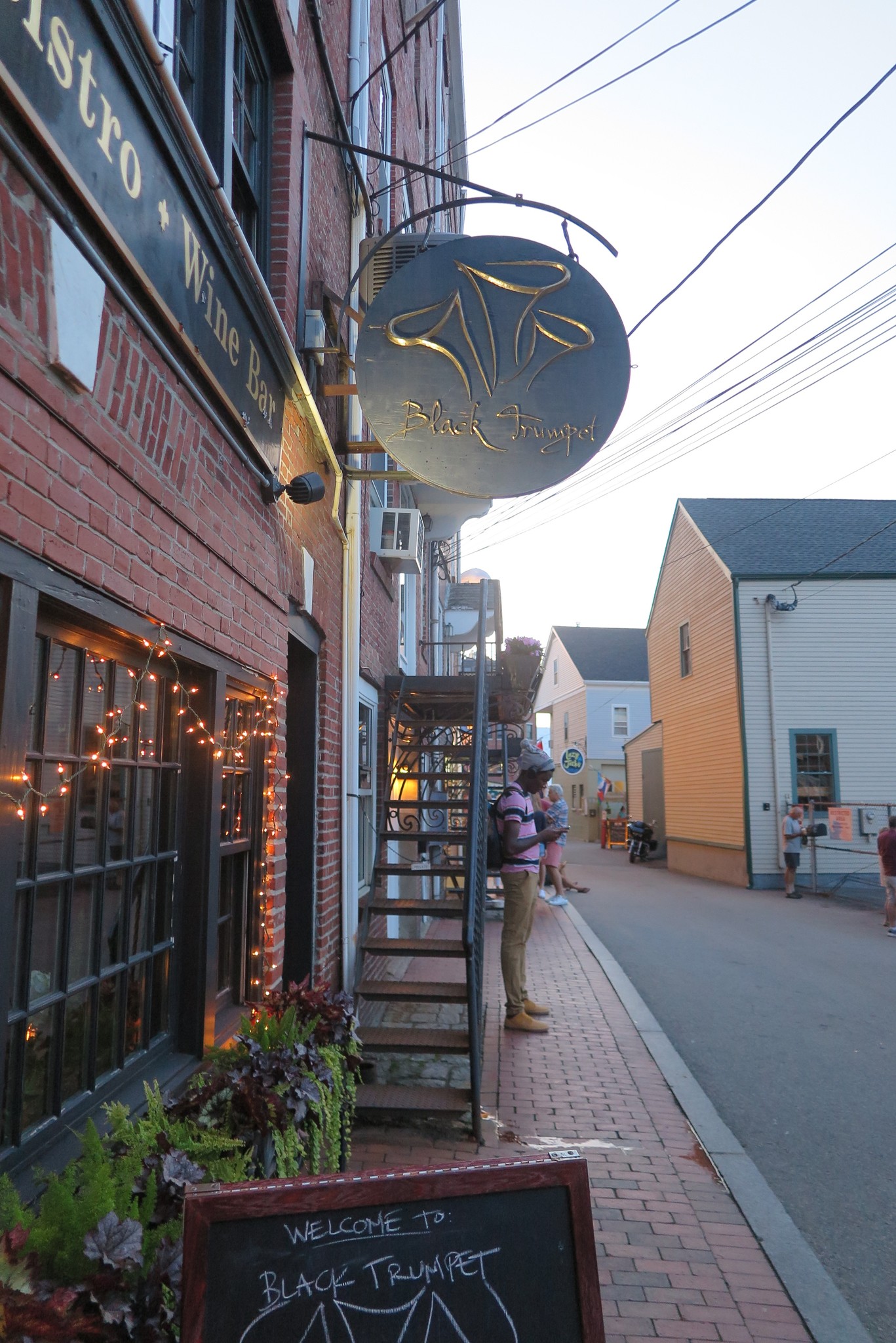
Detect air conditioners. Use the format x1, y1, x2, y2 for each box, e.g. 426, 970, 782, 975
359, 234, 467, 322
369, 507, 425, 574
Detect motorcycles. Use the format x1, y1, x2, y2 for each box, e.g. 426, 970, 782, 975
627, 815, 660, 863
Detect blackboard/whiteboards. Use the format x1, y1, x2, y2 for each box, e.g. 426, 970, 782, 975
178, 1149, 606, 1343
607, 820, 628, 845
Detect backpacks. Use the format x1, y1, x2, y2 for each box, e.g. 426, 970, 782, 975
486, 786, 528, 869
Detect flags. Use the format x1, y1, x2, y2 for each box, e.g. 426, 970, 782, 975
596, 772, 612, 804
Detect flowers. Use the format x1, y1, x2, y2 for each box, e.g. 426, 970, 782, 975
505, 636, 545, 656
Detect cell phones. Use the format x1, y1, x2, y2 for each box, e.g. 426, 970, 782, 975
564, 825, 570, 829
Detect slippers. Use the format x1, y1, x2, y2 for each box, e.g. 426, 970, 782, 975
566, 881, 578, 891
578, 887, 590, 893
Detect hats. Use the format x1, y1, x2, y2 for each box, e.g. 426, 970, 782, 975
520, 739, 555, 773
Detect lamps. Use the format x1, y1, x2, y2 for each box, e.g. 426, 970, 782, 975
261, 472, 325, 505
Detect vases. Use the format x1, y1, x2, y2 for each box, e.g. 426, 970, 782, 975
505, 654, 541, 690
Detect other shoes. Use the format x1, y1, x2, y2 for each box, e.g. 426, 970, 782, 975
883, 919, 891, 928
504, 1011, 549, 1033
538, 888, 545, 898
522, 998, 551, 1015
887, 926, 896, 937
544, 894, 568, 906
786, 891, 802, 899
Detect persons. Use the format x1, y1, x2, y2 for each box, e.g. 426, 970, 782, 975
530, 792, 548, 899
543, 849, 591, 892
878, 826, 890, 926
495, 739, 555, 1031
781, 806, 807, 899
878, 816, 896, 938
545, 783, 569, 906
